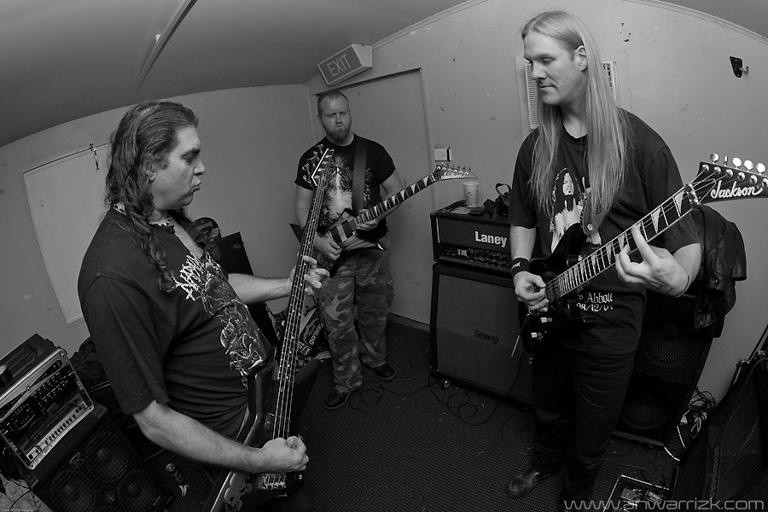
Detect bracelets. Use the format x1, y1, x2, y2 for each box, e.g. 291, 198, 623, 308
509, 257, 531, 278
671, 275, 690, 299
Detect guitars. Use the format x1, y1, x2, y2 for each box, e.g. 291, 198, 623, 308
200, 149, 355, 512
490, 154, 768, 378
288, 158, 478, 271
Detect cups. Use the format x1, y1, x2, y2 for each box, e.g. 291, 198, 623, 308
462, 181, 479, 208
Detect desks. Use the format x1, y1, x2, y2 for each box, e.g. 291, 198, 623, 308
429, 264, 712, 447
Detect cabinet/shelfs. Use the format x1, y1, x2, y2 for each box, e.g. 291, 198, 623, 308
18, 140, 112, 326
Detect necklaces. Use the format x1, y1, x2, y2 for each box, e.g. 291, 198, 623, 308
114, 203, 171, 227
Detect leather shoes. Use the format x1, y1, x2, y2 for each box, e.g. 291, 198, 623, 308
505, 465, 552, 498
366, 362, 397, 383
322, 387, 353, 410
556, 463, 602, 512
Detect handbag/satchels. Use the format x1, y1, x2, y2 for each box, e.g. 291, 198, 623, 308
496, 189, 510, 215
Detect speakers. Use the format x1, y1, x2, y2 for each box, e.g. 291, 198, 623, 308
671, 324, 768, 512
11, 399, 175, 511
221, 232, 278, 346
612, 293, 714, 449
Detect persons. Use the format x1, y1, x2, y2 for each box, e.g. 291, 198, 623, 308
293, 90, 407, 411
504, 9, 703, 512
76, 97, 330, 512
